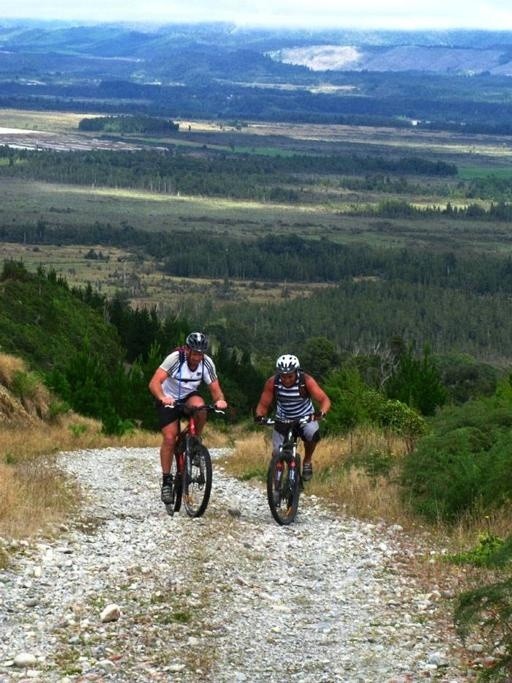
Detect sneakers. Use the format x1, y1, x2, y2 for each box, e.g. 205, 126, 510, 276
272, 490, 282, 508
161, 474, 173, 504
302, 459, 313, 481
192, 462, 205, 485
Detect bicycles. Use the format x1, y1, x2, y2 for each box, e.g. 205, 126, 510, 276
255, 411, 327, 526
153, 399, 232, 518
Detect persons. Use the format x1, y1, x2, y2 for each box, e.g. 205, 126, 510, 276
146, 330, 229, 505
254, 353, 332, 506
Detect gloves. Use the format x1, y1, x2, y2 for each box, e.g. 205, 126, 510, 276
257, 415, 268, 426
306, 412, 326, 422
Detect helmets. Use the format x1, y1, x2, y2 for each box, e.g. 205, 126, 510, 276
275, 354, 300, 375
185, 331, 209, 354
